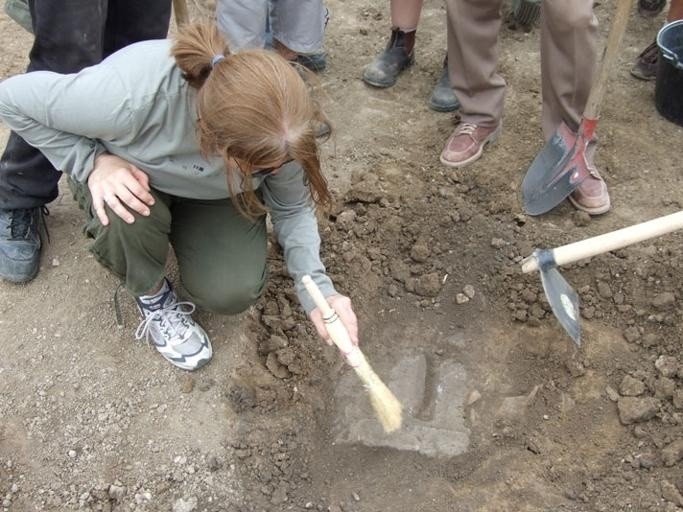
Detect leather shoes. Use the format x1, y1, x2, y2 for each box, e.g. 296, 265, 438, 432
439, 116, 503, 168
567, 162, 611, 217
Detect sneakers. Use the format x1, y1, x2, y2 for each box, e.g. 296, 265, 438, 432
132, 276, 212, 371
0, 204, 49, 283
631, 37, 659, 80
294, 53, 327, 71
638, 0, 666, 18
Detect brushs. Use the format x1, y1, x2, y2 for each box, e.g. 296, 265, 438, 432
300, 275, 404, 434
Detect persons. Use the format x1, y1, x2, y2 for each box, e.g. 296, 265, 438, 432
437, 1, 612, 217
0, 1, 359, 372
0, 1, 173, 288
360, 2, 462, 113
212, 1, 329, 76
625, 0, 683, 83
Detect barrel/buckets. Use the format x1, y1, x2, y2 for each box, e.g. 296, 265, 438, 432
654, 19, 682, 123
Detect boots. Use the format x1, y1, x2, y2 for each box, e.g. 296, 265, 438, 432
429, 51, 462, 112
362, 27, 417, 88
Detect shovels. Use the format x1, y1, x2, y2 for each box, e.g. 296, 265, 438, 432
519, 0, 634, 218
